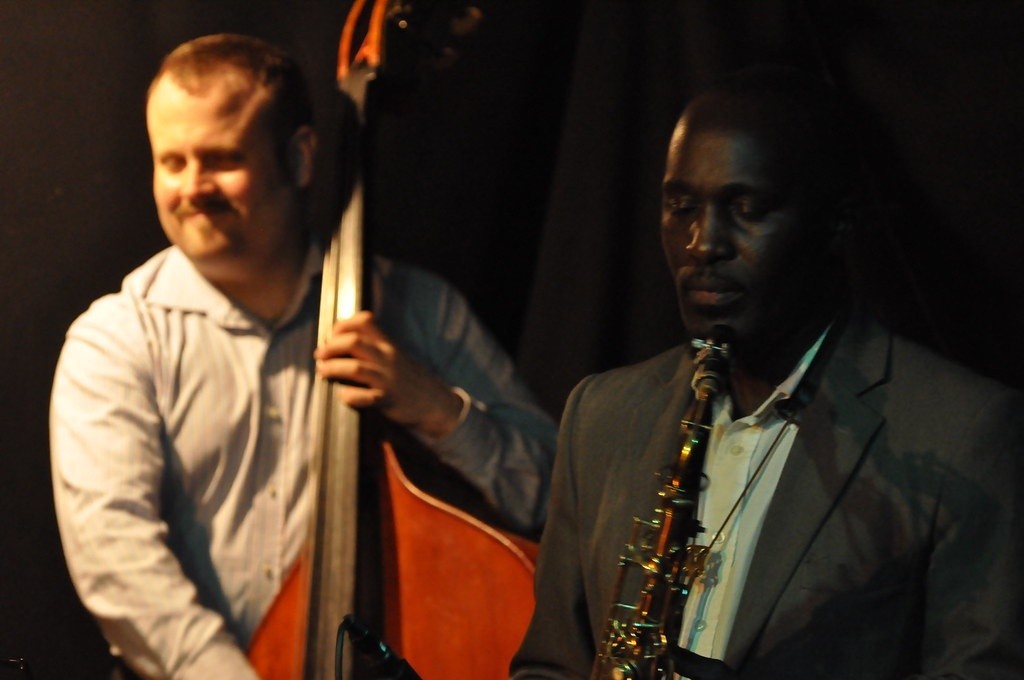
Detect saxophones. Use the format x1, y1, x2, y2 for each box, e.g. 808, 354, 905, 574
588, 321, 747, 680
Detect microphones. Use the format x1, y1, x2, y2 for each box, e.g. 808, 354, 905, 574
342, 613, 425, 680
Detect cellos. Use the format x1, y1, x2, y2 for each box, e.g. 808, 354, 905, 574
243, 0, 541, 680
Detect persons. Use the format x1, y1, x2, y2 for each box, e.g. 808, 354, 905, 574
49, 34, 559, 680
504, 74, 1024, 680
